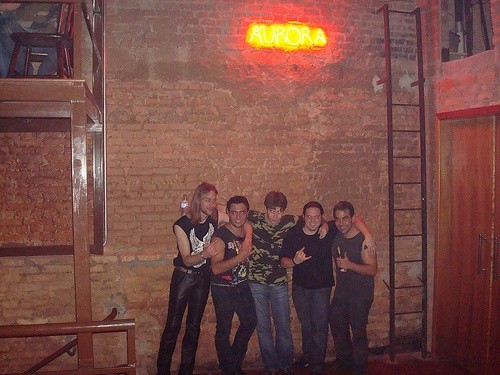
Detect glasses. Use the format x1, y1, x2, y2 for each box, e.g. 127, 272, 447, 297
229, 211, 248, 215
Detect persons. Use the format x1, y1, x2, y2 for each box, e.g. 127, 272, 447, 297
156, 182, 378, 375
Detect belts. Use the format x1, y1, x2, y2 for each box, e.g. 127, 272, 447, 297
211, 278, 247, 288
175, 265, 210, 275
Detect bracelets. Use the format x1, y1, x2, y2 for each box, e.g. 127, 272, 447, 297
200, 253, 206, 260
292, 257, 297, 266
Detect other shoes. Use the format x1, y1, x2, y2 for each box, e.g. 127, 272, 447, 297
268, 368, 294, 375
329, 361, 348, 371
352, 368, 363, 375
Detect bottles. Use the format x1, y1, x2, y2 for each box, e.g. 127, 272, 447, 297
180, 194, 189, 216
337, 246, 347, 271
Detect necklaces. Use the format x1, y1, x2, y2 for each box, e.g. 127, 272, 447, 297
230, 225, 243, 239
